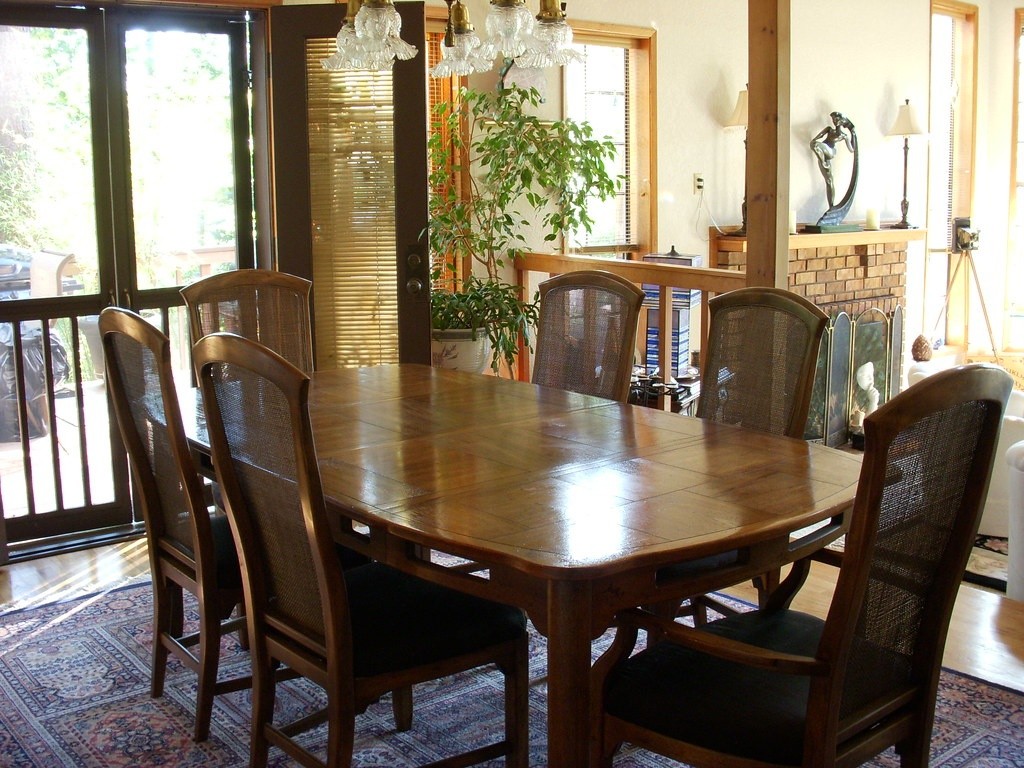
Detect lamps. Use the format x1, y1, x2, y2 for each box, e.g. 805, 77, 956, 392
883, 97, 927, 230
316, 0, 579, 79
723, 84, 748, 236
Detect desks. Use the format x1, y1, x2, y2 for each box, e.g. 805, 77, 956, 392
120, 365, 865, 767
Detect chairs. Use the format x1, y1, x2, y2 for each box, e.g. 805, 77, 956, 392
589, 364, 1012, 768
180, 267, 315, 374
531, 268, 647, 406
98, 306, 312, 740
190, 333, 530, 768
672, 285, 829, 630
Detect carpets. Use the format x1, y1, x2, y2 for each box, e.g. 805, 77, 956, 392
0, 577, 1024, 768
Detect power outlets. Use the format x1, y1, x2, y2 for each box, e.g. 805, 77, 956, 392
693, 173, 705, 195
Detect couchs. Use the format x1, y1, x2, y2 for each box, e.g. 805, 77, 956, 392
907, 365, 1024, 538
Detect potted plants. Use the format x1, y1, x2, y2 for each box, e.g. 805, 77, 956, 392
430, 274, 537, 376
